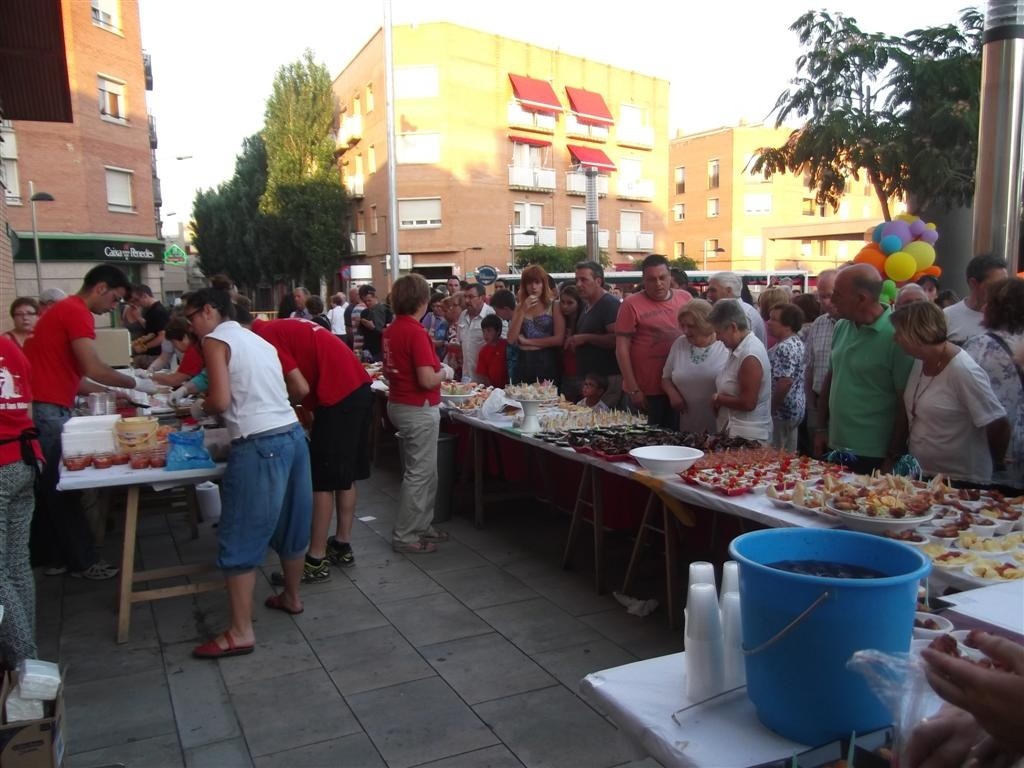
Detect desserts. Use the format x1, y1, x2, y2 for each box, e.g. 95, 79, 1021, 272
830, 473, 1024, 630
440, 379, 494, 410
504, 375, 558, 399
534, 395, 761, 456
684, 446, 850, 508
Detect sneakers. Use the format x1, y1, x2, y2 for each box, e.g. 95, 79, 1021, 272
39, 562, 66, 576
270, 555, 334, 587
72, 559, 119, 580
326, 536, 356, 568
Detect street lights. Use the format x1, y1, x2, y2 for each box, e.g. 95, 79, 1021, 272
463, 246, 482, 281
27, 180, 54, 297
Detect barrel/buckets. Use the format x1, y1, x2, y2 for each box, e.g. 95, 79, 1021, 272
113, 415, 159, 452
728, 526, 936, 749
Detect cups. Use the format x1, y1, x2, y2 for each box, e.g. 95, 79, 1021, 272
683, 561, 746, 705
88, 392, 116, 415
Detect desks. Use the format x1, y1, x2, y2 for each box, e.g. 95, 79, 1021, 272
56, 416, 230, 643
439, 404, 1024, 768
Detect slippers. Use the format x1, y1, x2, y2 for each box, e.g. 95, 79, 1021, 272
264, 594, 304, 614
192, 632, 253, 658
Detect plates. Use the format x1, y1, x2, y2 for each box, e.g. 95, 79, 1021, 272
680, 458, 851, 523
881, 531, 1024, 584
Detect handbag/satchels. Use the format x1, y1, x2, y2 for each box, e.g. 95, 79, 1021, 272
164, 431, 216, 470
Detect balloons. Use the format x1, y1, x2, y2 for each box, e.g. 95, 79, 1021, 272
852, 213, 941, 302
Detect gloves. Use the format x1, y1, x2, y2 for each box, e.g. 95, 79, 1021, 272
167, 387, 187, 406
441, 363, 454, 380
135, 378, 156, 393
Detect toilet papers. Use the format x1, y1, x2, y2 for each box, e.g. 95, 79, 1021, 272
196, 483, 222, 518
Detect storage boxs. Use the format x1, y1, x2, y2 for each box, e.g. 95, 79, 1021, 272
0, 664, 70, 768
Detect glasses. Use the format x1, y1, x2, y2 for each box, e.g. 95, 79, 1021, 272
14, 311, 35, 318
464, 294, 480, 300
185, 309, 205, 325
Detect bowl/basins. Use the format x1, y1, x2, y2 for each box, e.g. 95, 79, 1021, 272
63, 449, 168, 470
440, 391, 477, 404
890, 489, 1024, 549
911, 609, 984, 664
629, 445, 705, 474
824, 497, 937, 535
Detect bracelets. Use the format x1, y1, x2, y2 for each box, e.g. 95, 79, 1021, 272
714, 392, 719, 402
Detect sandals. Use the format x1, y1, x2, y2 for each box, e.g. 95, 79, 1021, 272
395, 539, 437, 553
421, 527, 450, 543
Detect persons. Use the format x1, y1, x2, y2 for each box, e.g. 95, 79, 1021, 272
186, 275, 312, 658
23, 264, 158, 581
4, 298, 40, 349
0, 333, 47, 670
35, 255, 1024, 584
906, 628, 1024, 768
